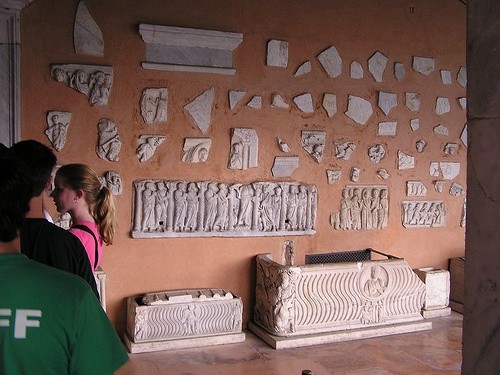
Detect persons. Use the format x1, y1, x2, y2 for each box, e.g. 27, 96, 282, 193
0, 143, 136, 375
11, 139, 113, 304
45, 68, 462, 232
